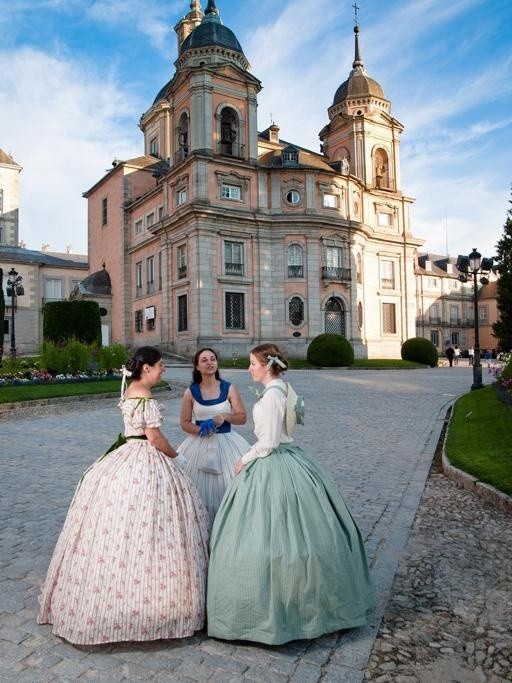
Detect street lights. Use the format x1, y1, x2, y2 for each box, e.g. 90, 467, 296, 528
468, 248, 486, 390
7, 266, 18, 358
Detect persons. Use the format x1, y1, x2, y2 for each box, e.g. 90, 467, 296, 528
172, 346, 254, 528
445, 343, 456, 367
467, 345, 474, 366
484, 348, 493, 368
204, 342, 374, 645
34, 344, 212, 648
453, 344, 462, 365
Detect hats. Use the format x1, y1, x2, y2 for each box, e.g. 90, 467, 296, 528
283, 381, 307, 439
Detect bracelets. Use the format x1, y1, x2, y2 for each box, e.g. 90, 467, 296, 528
216, 413, 224, 423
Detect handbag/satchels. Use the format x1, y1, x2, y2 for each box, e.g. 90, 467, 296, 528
196, 428, 224, 474
